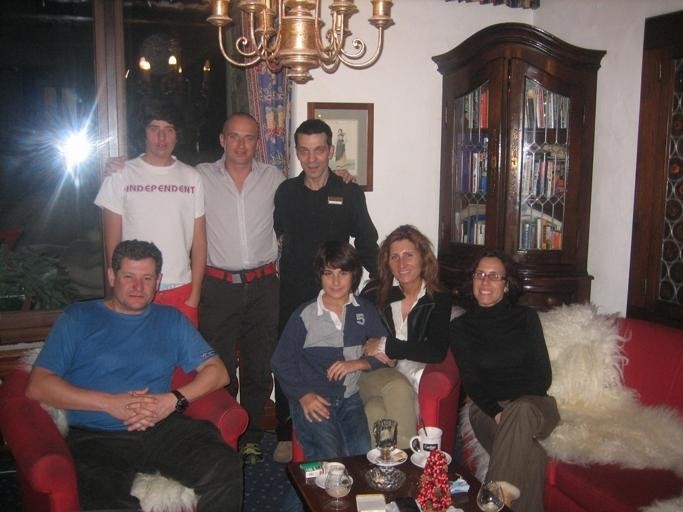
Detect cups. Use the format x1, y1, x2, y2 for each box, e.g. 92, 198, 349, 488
322, 462, 346, 484
410, 426, 443, 460
475, 481, 505, 512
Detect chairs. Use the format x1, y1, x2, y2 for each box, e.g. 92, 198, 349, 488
437, 318, 683, 512
290, 345, 460, 463
1, 350, 249, 511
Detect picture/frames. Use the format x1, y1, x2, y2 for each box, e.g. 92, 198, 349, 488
307, 102, 375, 192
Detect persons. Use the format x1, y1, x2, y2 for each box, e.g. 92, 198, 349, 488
451, 249, 560, 512
272, 118, 380, 443
357, 224, 451, 455
335, 129, 347, 167
103, 110, 359, 427
88, 102, 208, 330
24, 239, 243, 512
269, 238, 398, 460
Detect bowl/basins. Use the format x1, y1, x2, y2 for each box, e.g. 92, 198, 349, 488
365, 467, 407, 492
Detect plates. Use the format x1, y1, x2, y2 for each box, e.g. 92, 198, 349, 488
315, 475, 354, 490
410, 451, 452, 469
366, 447, 408, 467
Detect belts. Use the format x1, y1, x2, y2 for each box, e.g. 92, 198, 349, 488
205, 261, 276, 285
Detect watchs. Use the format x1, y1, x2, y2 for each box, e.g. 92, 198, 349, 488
171, 389, 188, 413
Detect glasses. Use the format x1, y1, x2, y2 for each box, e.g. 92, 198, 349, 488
470, 270, 509, 284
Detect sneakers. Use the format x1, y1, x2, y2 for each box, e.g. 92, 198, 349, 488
238, 441, 263, 465
272, 438, 296, 464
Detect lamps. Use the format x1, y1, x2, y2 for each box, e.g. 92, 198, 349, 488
206, 0, 396, 85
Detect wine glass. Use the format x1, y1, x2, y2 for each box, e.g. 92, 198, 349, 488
324, 469, 352, 511
372, 419, 398, 463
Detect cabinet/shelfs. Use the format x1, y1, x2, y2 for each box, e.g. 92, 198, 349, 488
429, 23, 608, 314
